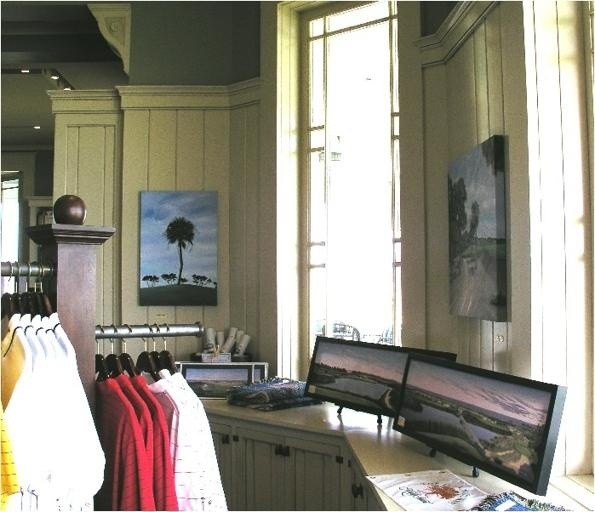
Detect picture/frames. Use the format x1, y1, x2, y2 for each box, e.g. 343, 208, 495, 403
391, 354, 569, 497
306, 336, 456, 421
174, 360, 269, 400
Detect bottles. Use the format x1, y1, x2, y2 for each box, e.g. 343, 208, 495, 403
206, 327, 250, 355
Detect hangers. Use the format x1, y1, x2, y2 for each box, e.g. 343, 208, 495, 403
95, 323, 180, 384
0, 260, 54, 325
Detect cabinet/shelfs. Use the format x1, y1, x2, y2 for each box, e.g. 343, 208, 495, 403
206, 413, 382, 511
